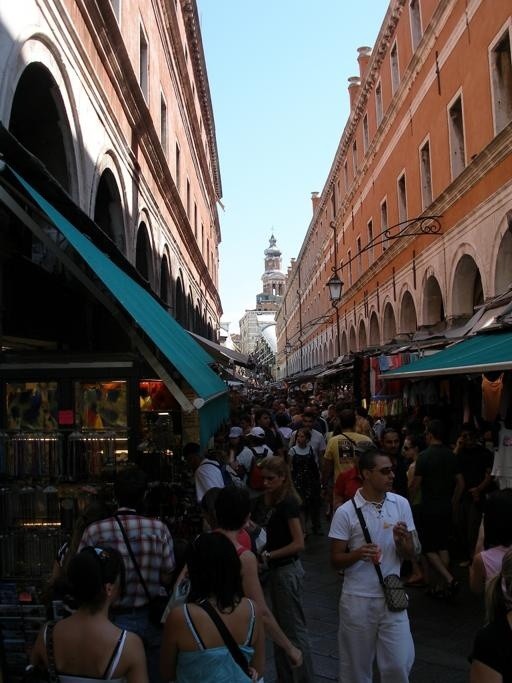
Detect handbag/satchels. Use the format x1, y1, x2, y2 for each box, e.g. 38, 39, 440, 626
383, 574, 409, 611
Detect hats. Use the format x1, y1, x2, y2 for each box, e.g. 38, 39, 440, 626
245, 427, 265, 439
229, 427, 242, 438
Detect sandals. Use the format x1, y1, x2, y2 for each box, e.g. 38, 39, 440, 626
443, 579, 461, 594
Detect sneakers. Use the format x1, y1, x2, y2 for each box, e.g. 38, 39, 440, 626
312, 529, 325, 536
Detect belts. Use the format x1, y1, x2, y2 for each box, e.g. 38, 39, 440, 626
277, 557, 299, 566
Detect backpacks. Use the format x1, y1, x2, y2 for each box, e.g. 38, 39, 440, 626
246, 447, 268, 489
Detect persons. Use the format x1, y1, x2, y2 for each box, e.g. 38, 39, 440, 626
468, 546, 512, 683
328, 450, 422, 683
76, 471, 177, 637
159, 534, 264, 683
68, 391, 512, 606
29, 547, 150, 683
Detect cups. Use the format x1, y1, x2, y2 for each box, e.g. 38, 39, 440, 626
372, 542, 384, 564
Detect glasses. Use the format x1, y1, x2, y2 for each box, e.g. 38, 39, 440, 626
304, 420, 312, 422
371, 466, 395, 474
386, 439, 413, 450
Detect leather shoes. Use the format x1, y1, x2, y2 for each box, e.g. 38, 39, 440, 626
403, 579, 427, 589
